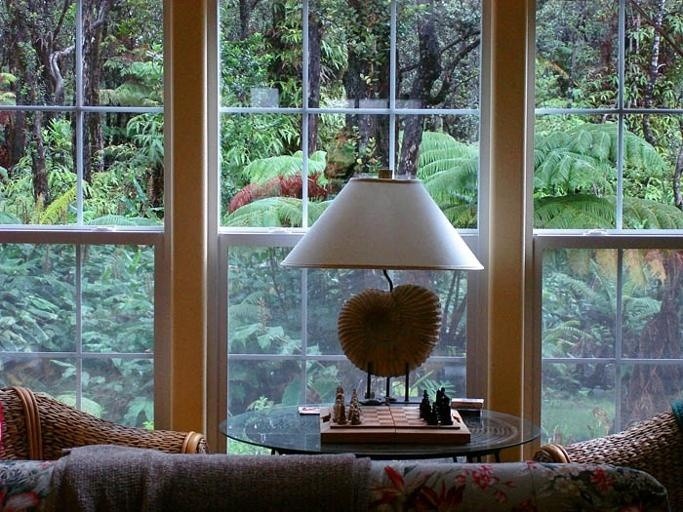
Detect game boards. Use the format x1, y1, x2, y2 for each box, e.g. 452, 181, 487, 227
330, 406, 461, 429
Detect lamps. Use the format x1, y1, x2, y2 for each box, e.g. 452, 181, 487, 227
278, 168, 485, 408
